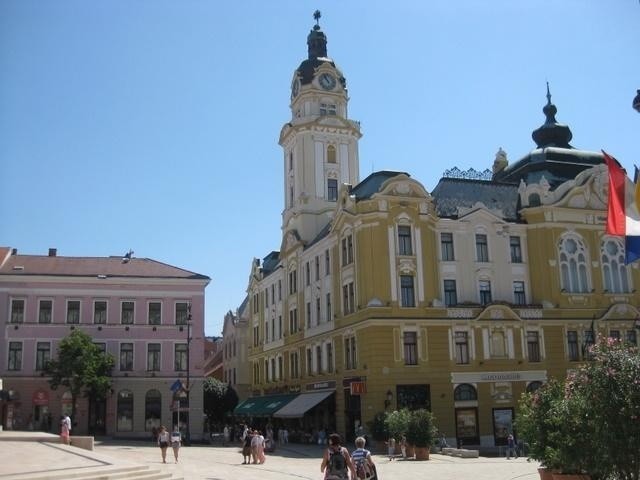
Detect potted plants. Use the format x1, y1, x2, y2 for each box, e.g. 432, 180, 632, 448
410, 408, 438, 460
382, 409, 409, 462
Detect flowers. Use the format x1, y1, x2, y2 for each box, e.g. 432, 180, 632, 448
515, 331, 640, 405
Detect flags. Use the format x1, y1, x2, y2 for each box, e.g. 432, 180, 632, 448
602, 149, 640, 236
623, 162, 640, 266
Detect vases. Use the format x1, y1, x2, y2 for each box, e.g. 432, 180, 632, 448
535, 465, 553, 480
554, 460, 591, 480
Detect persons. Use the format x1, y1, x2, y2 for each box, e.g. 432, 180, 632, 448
152, 419, 335, 465
321, 433, 357, 480
350, 436, 378, 480
12, 413, 72, 445
385, 431, 447, 460
506, 434, 518, 460
